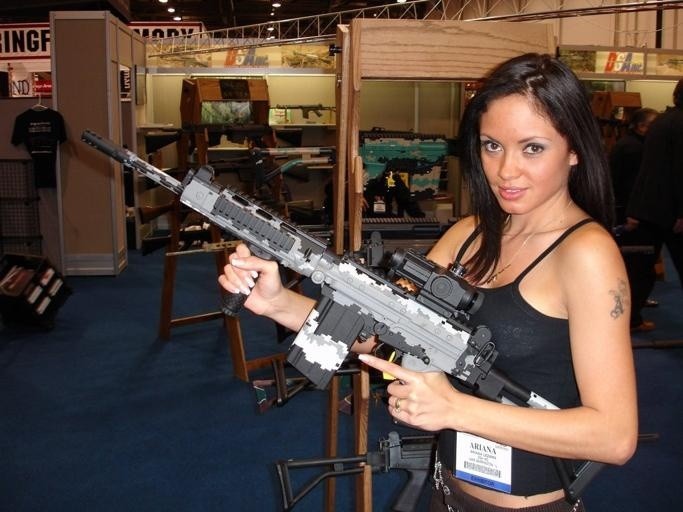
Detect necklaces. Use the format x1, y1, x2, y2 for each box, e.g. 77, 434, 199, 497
485, 199, 574, 285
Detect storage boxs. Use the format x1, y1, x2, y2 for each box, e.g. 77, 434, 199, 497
0, 248, 73, 332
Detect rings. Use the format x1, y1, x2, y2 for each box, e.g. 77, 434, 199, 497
394, 398, 402, 411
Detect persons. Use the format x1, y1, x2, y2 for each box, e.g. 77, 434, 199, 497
219, 52, 639, 509
610, 79, 683, 332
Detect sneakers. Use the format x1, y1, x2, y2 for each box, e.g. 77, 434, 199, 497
643, 293, 661, 309
630, 318, 658, 335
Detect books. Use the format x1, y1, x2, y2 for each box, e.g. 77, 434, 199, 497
0, 259, 63, 314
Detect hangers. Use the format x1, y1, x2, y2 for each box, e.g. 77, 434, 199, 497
26, 92, 48, 112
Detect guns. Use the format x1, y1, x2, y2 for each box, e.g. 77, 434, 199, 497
78, 130, 605, 508
356, 124, 462, 217
250, 365, 392, 417
273, 434, 439, 512
138, 103, 339, 255
166, 216, 465, 257
